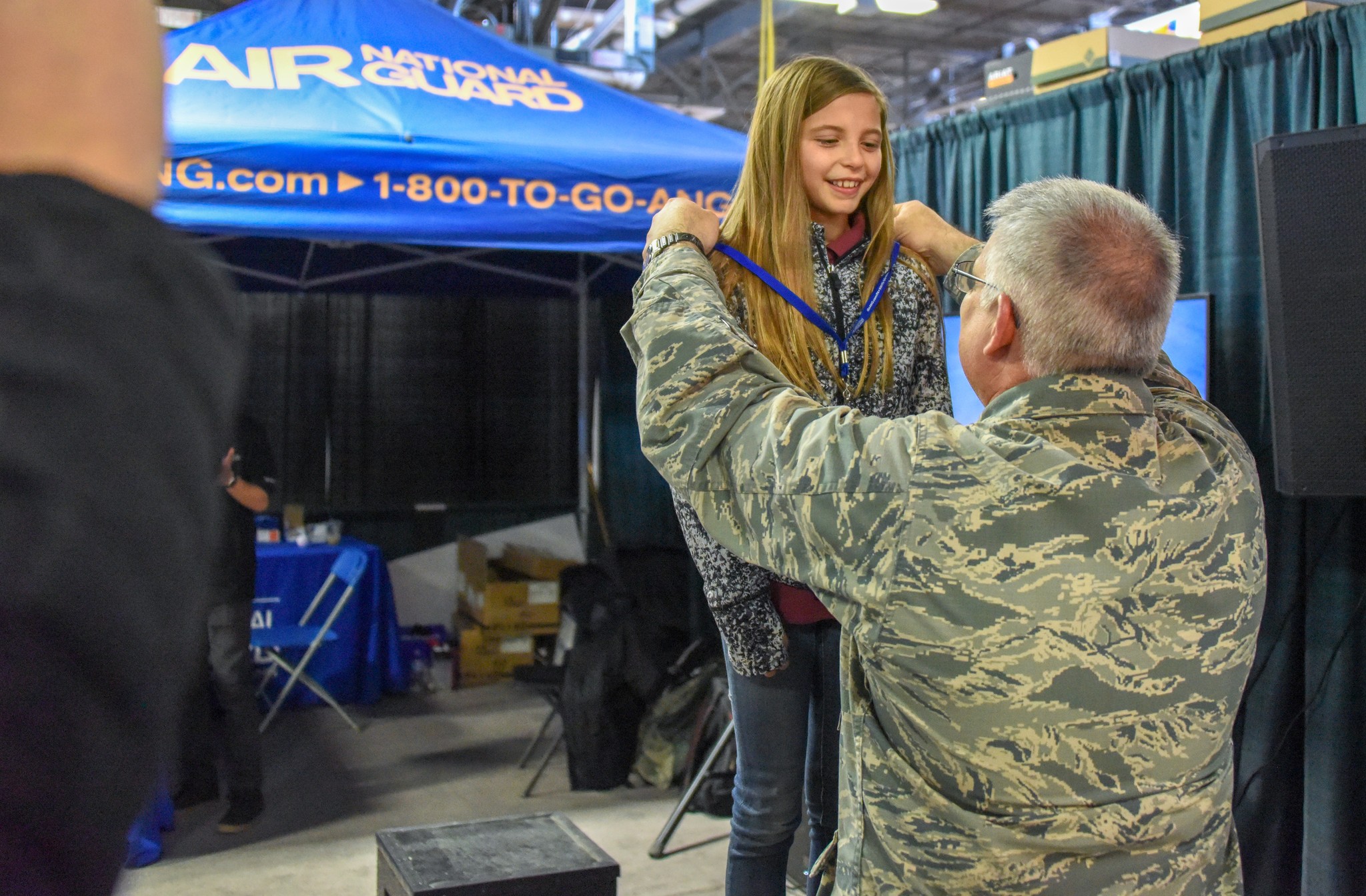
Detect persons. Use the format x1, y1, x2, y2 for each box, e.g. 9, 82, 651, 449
621, 173, 1276, 894
641, 51, 954, 896
0, 0, 169, 896
159, 384, 270, 837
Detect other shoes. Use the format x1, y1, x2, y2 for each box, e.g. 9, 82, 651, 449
216, 801, 268, 832
178, 783, 221, 812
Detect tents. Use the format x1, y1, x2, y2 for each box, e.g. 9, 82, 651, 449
129, 3, 759, 255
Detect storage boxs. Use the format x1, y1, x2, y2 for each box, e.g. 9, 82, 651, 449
457, 536, 572, 638
452, 606, 538, 689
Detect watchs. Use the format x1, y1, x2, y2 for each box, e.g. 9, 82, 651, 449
640, 231, 707, 275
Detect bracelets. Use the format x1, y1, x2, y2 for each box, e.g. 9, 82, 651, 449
225, 473, 237, 489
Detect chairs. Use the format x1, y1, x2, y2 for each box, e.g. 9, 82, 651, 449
249, 544, 369, 737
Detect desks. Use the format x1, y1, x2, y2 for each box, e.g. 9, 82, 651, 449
246, 536, 411, 705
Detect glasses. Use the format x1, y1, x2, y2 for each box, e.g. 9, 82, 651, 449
951, 261, 1022, 328
